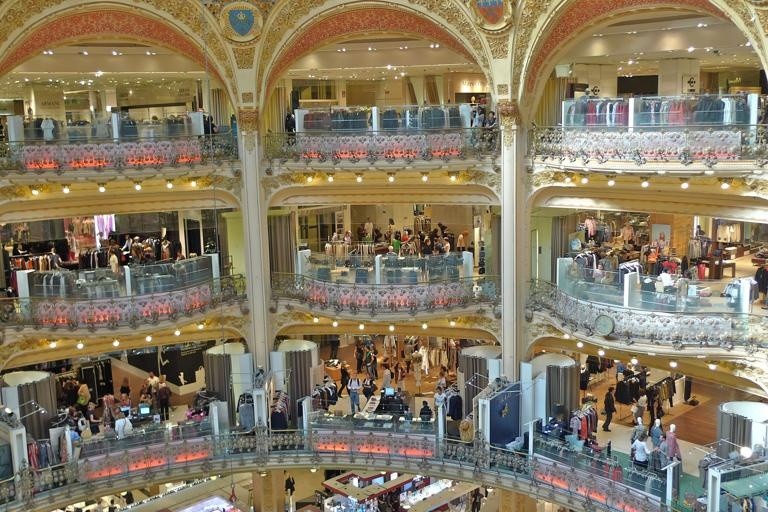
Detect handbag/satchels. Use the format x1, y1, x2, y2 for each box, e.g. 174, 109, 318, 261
630, 404, 638, 413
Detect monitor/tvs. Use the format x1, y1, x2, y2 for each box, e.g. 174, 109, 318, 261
386, 387, 395, 397
141, 407, 150, 414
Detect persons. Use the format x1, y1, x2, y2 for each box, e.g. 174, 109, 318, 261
472, 490, 484, 512
327, 334, 457, 422
696, 224, 705, 235
657, 232, 669, 251
107, 500, 118, 512
286, 474, 295, 495
204, 115, 219, 134
65, 370, 177, 442
643, 268, 673, 293
123, 490, 134, 504
601, 362, 681, 466
329, 217, 469, 259
592, 263, 606, 283
6, 233, 185, 272
95, 501, 103, 512
471, 106, 496, 150
622, 222, 634, 244
285, 113, 294, 140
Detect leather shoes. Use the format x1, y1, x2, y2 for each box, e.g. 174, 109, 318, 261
601, 425, 611, 432
